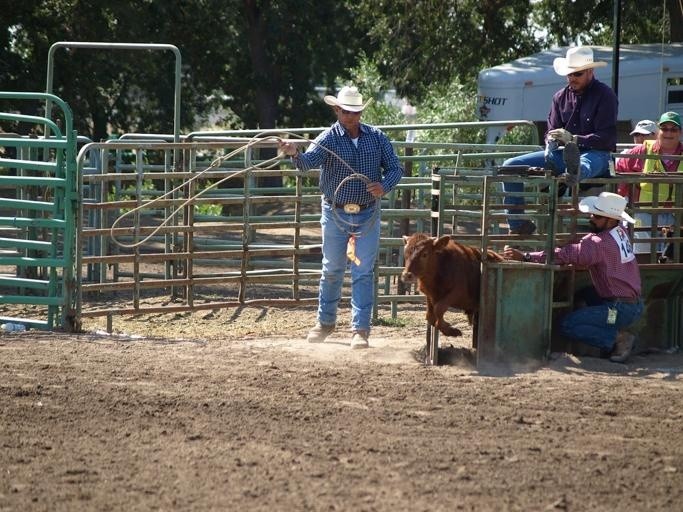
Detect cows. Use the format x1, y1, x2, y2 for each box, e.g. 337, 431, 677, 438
400, 231, 508, 338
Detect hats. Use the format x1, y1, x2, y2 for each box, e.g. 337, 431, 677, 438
630, 119, 659, 137
578, 192, 636, 224
324, 86, 374, 112
660, 111, 681, 127
552, 46, 608, 77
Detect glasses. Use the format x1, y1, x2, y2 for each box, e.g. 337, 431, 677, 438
567, 72, 584, 77
633, 132, 649, 137
659, 127, 680, 133
341, 109, 362, 115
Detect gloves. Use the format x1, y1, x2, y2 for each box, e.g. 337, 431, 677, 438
544, 127, 579, 163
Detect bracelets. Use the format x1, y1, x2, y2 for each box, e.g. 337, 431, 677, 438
291, 150, 297, 157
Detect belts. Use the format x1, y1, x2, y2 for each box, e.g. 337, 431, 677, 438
602, 295, 641, 305
324, 198, 377, 214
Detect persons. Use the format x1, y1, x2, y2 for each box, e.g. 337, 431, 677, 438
614, 119, 657, 167
498, 191, 646, 365
277, 84, 404, 349
612, 110, 683, 253
501, 46, 619, 249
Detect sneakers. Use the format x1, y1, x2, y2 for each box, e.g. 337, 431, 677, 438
350, 329, 369, 350
563, 141, 582, 186
611, 332, 636, 362
308, 322, 335, 343
503, 221, 538, 251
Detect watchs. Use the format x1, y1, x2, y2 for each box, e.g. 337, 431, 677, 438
522, 248, 531, 262
